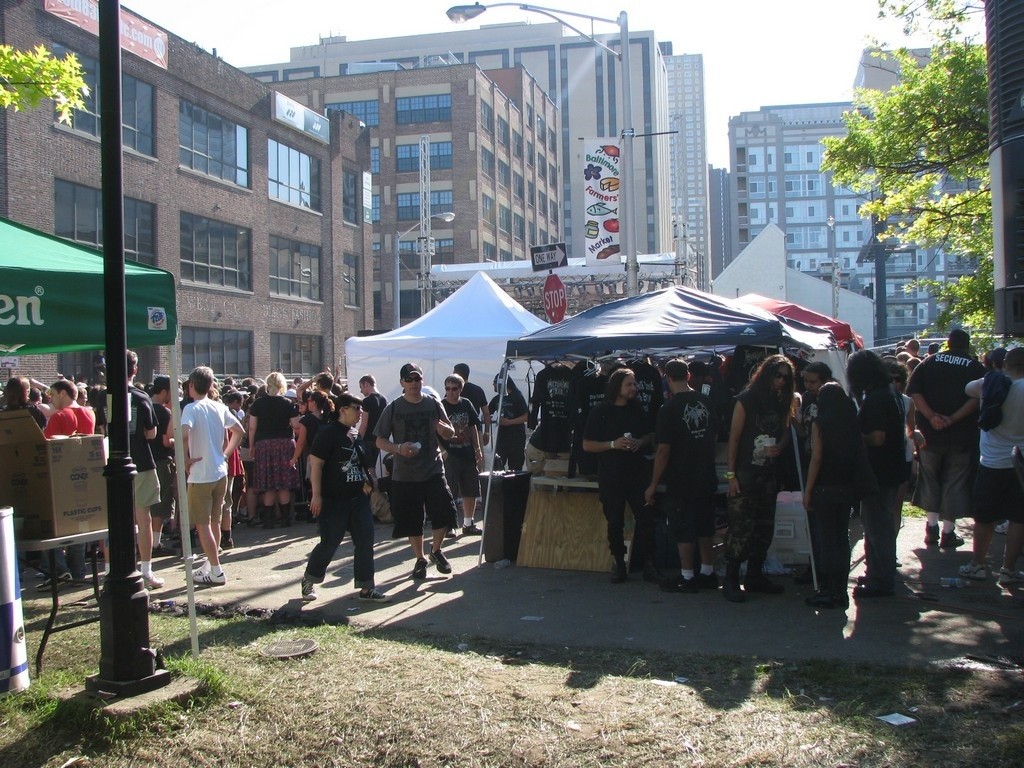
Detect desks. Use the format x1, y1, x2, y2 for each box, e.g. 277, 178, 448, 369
16, 524, 140, 679
516, 474, 637, 573
478, 470, 532, 563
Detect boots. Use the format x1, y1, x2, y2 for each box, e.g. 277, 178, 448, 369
639, 553, 668, 587
744, 560, 784, 593
610, 554, 625, 583
723, 566, 745, 601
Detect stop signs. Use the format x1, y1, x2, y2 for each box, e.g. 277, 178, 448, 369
542, 274, 567, 325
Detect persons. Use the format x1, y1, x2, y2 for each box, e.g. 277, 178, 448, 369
374, 362, 458, 580
300, 392, 392, 604
0, 329, 1024, 607
182, 366, 247, 584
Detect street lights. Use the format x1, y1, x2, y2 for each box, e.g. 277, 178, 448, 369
445, 1, 640, 301
392, 211, 455, 330
826, 214, 838, 319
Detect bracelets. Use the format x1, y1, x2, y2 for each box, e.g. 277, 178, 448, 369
474, 447, 481, 451
223, 453, 229, 460
441, 450, 447, 455
611, 441, 615, 449
397, 444, 401, 454
723, 471, 736, 479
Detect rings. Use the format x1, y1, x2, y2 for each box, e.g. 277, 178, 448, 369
408, 450, 411, 453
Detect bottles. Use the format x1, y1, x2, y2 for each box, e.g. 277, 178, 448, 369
939, 577, 971, 589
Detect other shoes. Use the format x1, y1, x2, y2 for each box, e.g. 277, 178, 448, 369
27, 500, 482, 609
795, 569, 895, 606
995, 519, 1009, 534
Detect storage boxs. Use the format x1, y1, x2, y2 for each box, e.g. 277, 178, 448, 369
0, 409, 108, 538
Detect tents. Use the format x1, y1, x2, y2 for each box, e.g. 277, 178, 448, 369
732, 291, 866, 348
0, 215, 199, 661
476, 283, 831, 592
340, 270, 551, 472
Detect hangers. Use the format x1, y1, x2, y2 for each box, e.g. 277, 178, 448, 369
626, 350, 645, 367
582, 357, 602, 378
524, 361, 537, 383
548, 354, 564, 370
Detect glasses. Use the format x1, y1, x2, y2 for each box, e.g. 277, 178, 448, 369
347, 405, 360, 411
889, 375, 902, 383
445, 386, 459, 391
775, 372, 788, 380
404, 377, 422, 383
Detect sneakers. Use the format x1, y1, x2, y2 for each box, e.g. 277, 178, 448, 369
694, 572, 718, 589
998, 566, 1024, 585
663, 574, 700, 593
940, 530, 964, 547
925, 523, 940, 543
959, 563, 987, 581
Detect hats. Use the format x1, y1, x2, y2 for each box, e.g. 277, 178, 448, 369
401, 363, 422, 379
947, 330, 970, 348
335, 392, 362, 410
285, 389, 297, 398
665, 358, 687, 376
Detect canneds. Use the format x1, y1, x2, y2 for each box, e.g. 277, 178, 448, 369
623, 432, 632, 449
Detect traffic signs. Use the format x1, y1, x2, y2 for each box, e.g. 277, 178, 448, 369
530, 242, 568, 272
